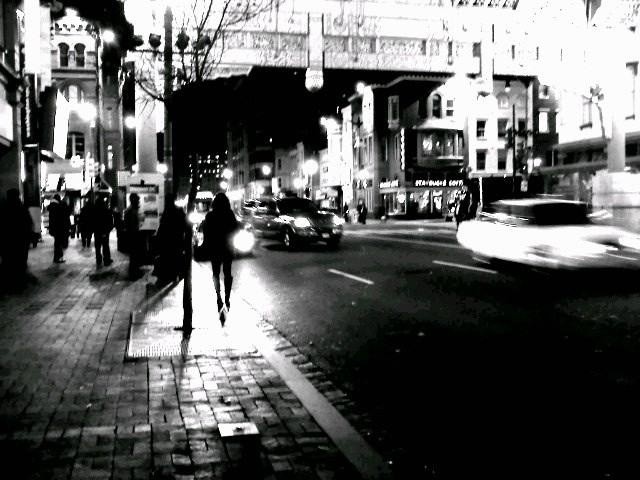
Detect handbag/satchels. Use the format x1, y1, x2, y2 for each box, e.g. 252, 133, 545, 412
193, 241, 210, 262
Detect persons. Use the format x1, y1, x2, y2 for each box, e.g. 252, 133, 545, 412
0, 190, 238, 312
343, 202, 368, 224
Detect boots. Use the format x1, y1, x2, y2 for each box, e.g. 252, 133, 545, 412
213, 275, 233, 311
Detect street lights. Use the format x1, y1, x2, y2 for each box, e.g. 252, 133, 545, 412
126, 8, 211, 194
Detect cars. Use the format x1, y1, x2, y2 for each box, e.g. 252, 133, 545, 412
193, 217, 256, 260
237, 195, 345, 249
455, 199, 639, 274
